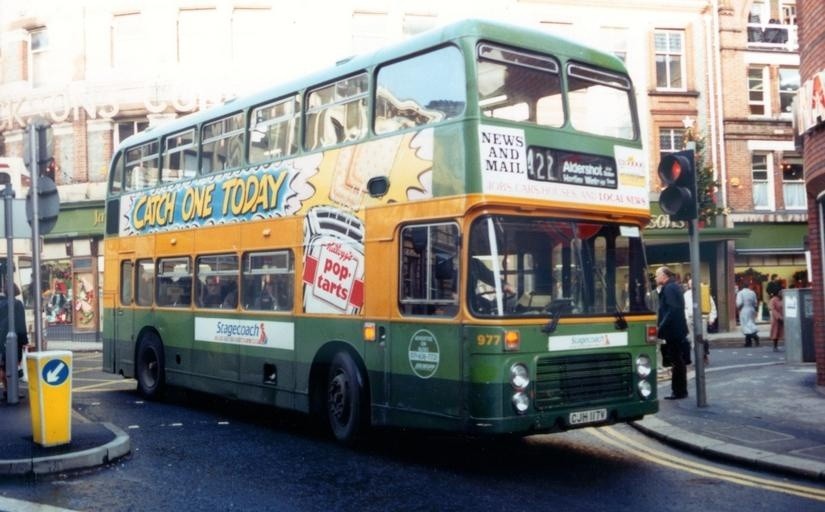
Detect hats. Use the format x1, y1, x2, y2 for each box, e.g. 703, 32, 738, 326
772, 287, 780, 295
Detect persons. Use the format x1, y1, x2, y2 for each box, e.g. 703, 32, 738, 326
119, 264, 291, 312
654, 266, 692, 399
736, 281, 761, 347
768, 287, 784, 351
433, 234, 520, 316
766, 273, 784, 300
623, 271, 658, 315
683, 276, 718, 368
0, 281, 29, 402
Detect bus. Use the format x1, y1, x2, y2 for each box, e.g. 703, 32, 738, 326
103, 18, 659, 443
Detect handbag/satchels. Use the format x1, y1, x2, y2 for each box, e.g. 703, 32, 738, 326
660, 341, 691, 366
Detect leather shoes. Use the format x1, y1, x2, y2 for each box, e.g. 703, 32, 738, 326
663, 392, 688, 400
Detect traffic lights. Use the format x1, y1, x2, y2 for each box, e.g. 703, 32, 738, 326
658, 149, 698, 221
